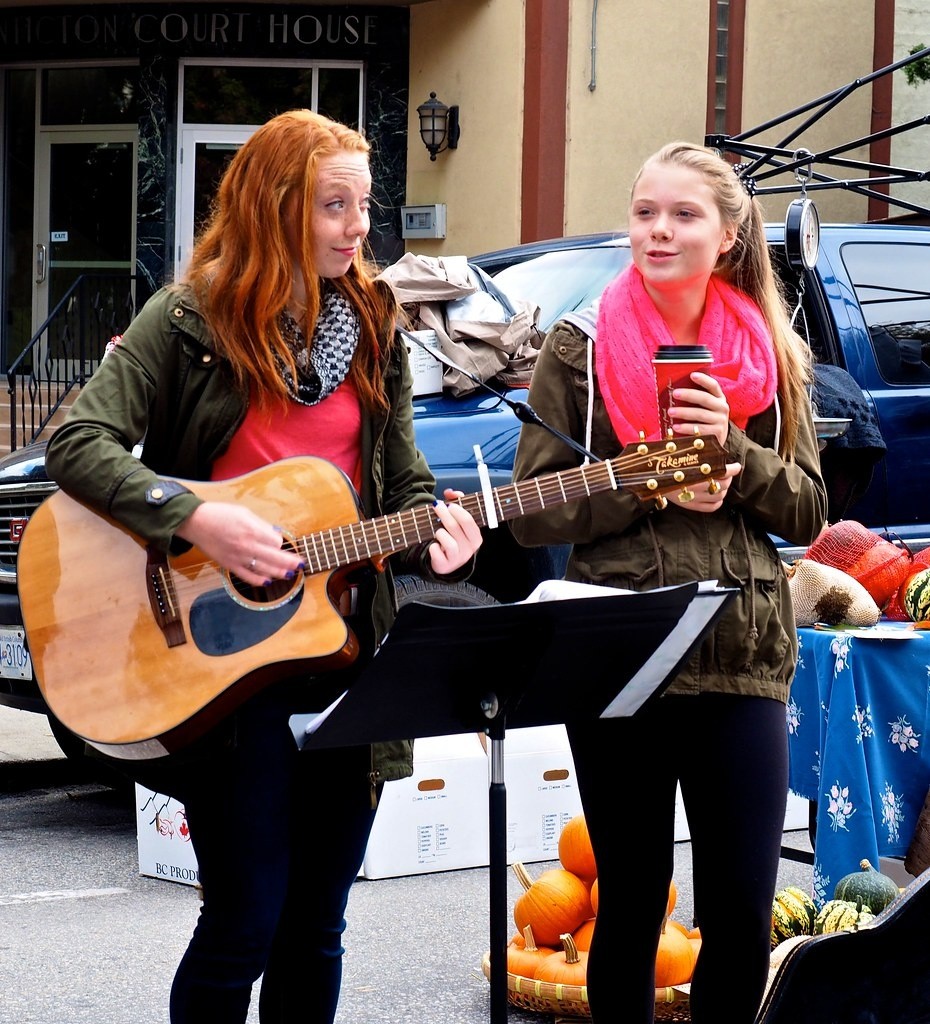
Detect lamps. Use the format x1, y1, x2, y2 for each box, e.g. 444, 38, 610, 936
416, 91, 460, 162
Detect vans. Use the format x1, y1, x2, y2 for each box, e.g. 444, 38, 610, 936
0, 217, 930, 790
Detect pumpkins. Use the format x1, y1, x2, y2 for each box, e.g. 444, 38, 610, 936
781, 519, 930, 629
769, 859, 906, 971
508, 813, 704, 987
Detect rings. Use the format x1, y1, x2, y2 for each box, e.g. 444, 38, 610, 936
250, 557, 256, 570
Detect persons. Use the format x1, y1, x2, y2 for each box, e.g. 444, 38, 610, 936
46, 108, 484, 1023
507, 142, 829, 1023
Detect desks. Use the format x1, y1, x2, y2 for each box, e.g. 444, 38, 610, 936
786, 620, 929, 912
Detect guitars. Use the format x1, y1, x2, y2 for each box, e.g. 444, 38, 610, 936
15, 426, 729, 762
